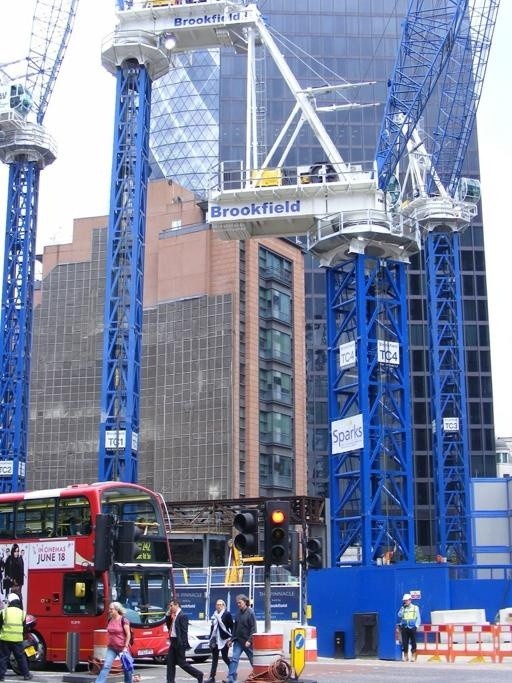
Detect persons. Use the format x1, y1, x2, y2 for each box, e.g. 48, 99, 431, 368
222, 593, 257, 683
203, 599, 234, 683
0, 544, 24, 610
431, 554, 446, 563
164, 598, 203, 683
382, 552, 396, 564
0, 593, 32, 682
398, 593, 421, 663
125, 585, 151, 613
95, 602, 134, 683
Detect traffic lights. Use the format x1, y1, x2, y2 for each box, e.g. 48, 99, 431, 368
265, 500, 290, 565
305, 536, 322, 569
234, 508, 259, 555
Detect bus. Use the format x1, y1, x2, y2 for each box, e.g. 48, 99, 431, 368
1, 480, 180, 666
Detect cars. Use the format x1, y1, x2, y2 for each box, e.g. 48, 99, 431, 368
152, 623, 212, 664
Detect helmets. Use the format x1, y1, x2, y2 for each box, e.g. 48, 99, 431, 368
8, 592, 21, 606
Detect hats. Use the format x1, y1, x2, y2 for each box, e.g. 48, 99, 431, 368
402, 594, 412, 601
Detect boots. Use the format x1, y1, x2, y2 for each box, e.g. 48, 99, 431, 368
403, 653, 409, 662
411, 652, 417, 661
204, 672, 216, 683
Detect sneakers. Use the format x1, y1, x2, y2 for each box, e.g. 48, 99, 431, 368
222, 677, 235, 683
24, 672, 33, 679
